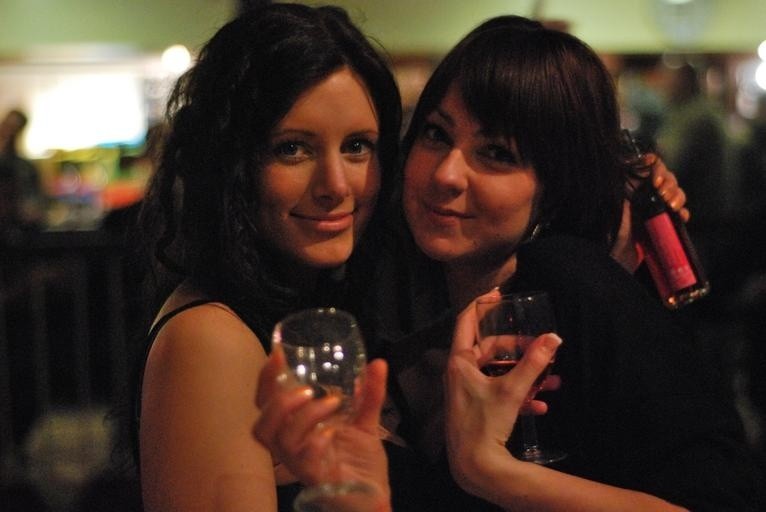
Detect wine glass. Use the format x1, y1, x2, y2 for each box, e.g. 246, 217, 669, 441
271, 307, 382, 512
476, 289, 569, 466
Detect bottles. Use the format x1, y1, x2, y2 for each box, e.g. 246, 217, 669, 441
616, 127, 713, 313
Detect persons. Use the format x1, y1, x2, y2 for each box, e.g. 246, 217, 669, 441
597, 38, 765, 329
361, 13, 766, 512
1, 108, 176, 387
129, 0, 404, 511
